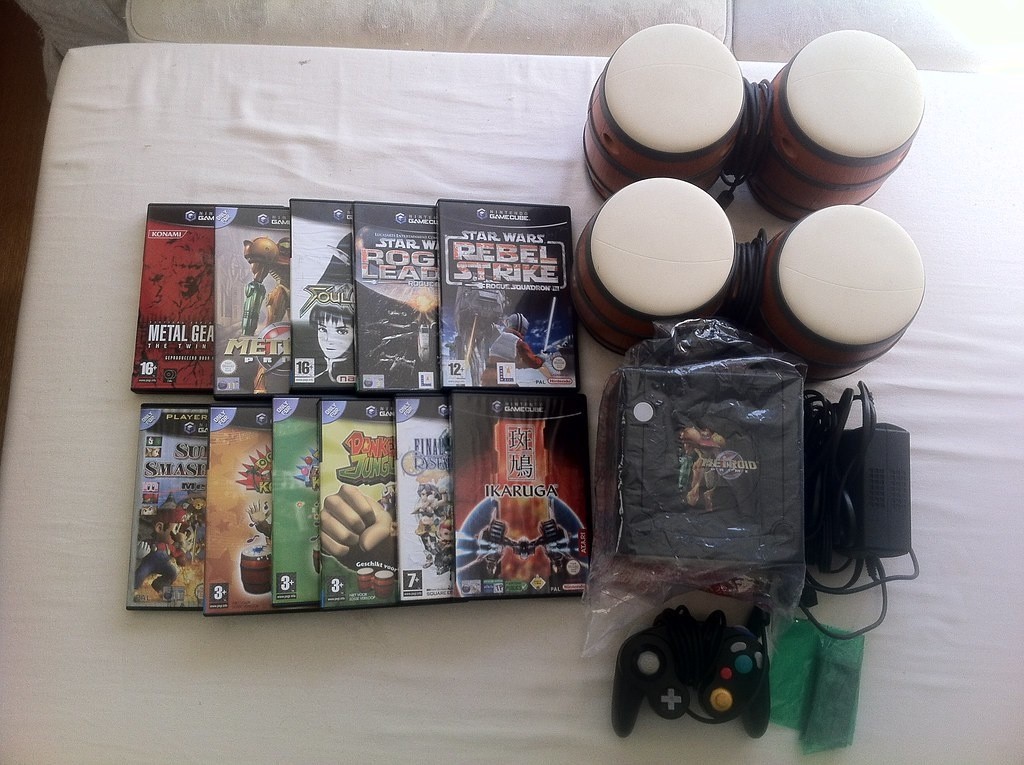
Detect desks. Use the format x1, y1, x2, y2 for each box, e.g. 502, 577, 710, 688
0, 40, 1024, 765
